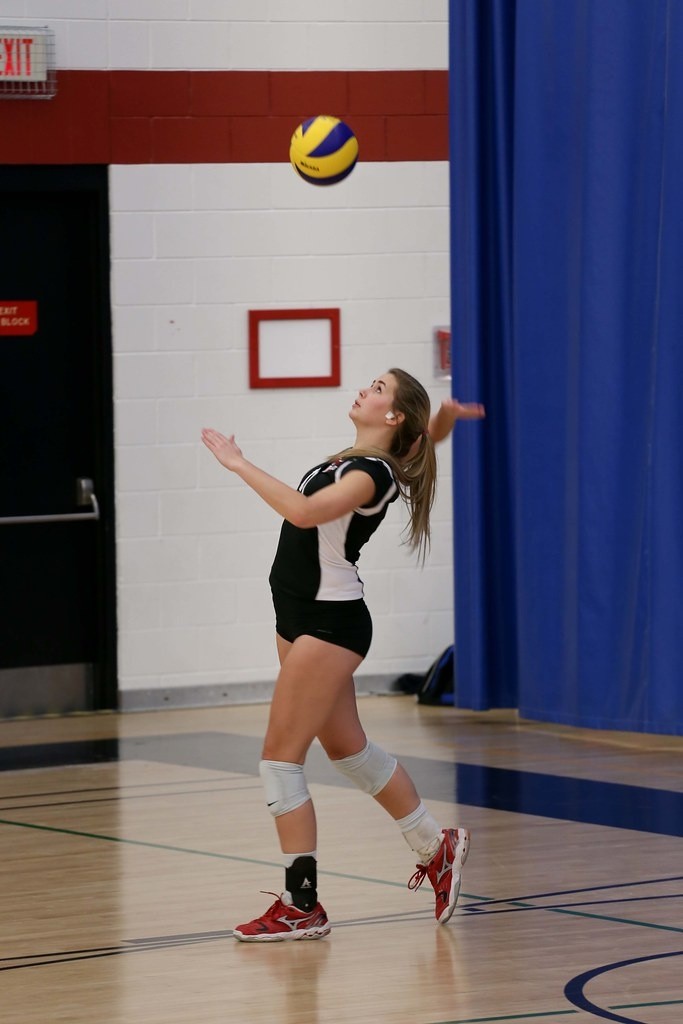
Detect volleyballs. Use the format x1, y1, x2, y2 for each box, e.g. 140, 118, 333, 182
288, 114, 360, 188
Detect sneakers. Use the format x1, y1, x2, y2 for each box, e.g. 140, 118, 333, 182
408, 827, 470, 925
232, 890, 332, 942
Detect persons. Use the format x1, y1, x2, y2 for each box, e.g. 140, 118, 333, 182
199, 368, 485, 940
